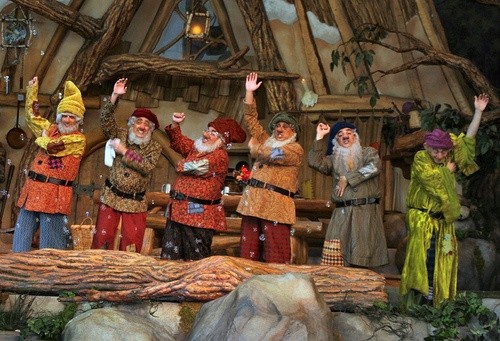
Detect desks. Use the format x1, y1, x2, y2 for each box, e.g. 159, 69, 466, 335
93, 190, 334, 264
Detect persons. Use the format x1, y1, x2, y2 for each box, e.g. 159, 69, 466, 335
162, 111, 238, 261
309, 120, 389, 270
11, 75, 87, 253
236, 71, 304, 265
402, 91, 490, 312
89, 76, 164, 253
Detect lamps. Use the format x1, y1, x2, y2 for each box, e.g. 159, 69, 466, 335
185, 1, 211, 41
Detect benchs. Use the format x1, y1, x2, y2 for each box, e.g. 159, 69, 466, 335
140, 215, 323, 264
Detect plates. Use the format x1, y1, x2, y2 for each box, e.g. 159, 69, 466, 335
225, 192, 243, 196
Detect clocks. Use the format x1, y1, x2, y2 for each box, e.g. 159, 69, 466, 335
1, 2, 33, 65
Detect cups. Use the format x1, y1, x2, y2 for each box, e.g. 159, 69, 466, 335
223, 186, 230, 195
161, 183, 171, 193
